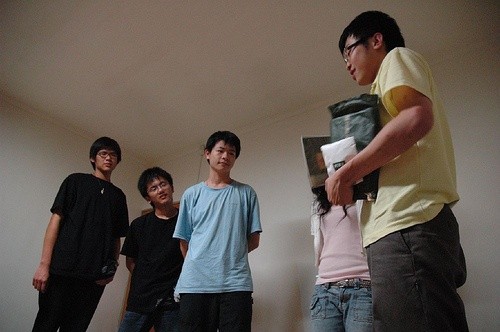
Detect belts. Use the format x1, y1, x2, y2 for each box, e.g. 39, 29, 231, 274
329, 279, 371, 288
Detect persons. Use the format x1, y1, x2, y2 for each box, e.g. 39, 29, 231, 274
119, 167, 179, 332
326, 11, 470, 332
310, 186, 372, 331
32, 137, 130, 332
173, 131, 262, 332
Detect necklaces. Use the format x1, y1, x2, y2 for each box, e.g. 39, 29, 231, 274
95, 174, 111, 195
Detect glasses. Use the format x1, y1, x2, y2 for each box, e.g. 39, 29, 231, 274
96, 152, 118, 158
146, 181, 169, 195
342, 38, 369, 63
